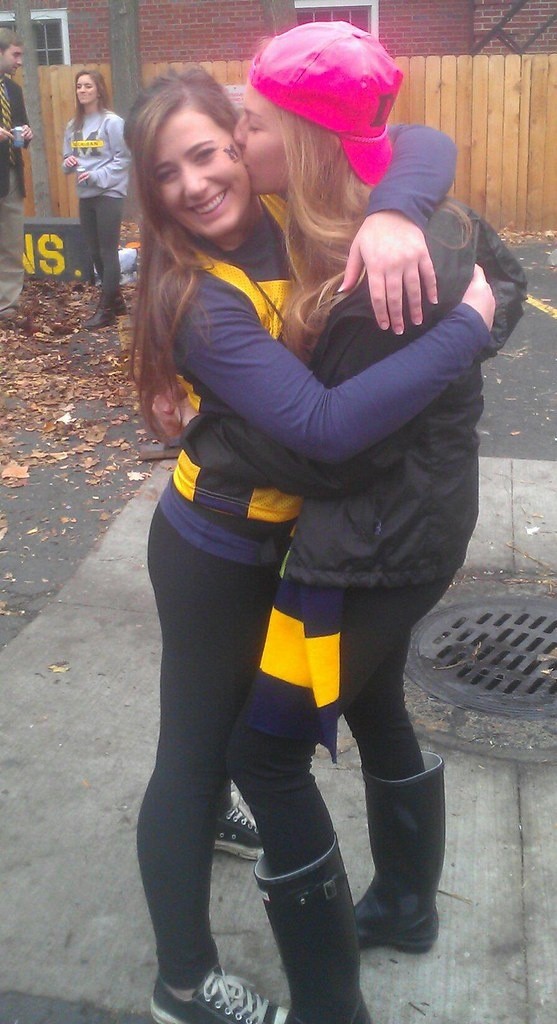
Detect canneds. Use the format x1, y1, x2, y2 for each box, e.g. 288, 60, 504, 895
14, 126, 24, 148
76, 166, 87, 187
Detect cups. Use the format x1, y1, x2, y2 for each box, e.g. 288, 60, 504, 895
76, 166, 88, 188
11, 127, 25, 147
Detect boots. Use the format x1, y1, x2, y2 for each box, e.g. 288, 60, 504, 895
81, 288, 116, 329
354, 750, 446, 954
114, 284, 127, 315
253, 832, 372, 1024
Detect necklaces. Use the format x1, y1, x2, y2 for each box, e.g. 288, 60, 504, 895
213, 194, 288, 327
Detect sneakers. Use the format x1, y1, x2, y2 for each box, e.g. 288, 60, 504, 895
150, 963, 290, 1024
213, 791, 265, 860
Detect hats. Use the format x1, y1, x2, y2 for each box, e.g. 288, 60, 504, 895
250, 21, 404, 186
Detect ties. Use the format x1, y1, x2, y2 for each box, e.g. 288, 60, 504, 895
0, 77, 16, 166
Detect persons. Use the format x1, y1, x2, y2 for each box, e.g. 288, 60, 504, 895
0, 27, 33, 321
62, 70, 132, 330
134, 21, 528, 1024
123, 67, 496, 1024
